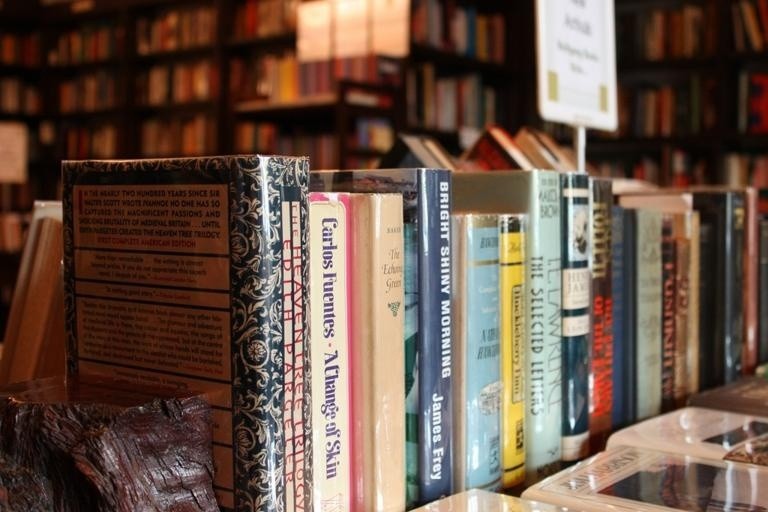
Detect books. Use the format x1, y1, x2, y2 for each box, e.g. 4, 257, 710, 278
0, 0, 767, 511
